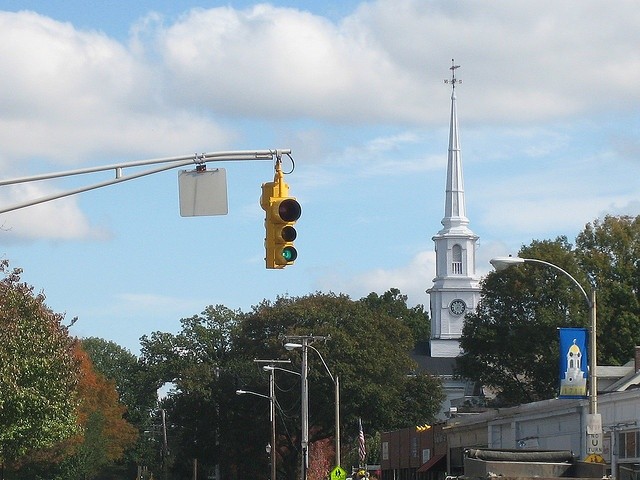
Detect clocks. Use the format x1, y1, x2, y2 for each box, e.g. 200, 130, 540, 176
448, 298, 467, 318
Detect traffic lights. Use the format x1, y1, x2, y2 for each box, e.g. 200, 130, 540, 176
258, 159, 301, 271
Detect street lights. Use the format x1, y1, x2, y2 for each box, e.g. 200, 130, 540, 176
490, 257, 606, 465
235, 388, 277, 479
282, 342, 346, 480
263, 365, 309, 479
